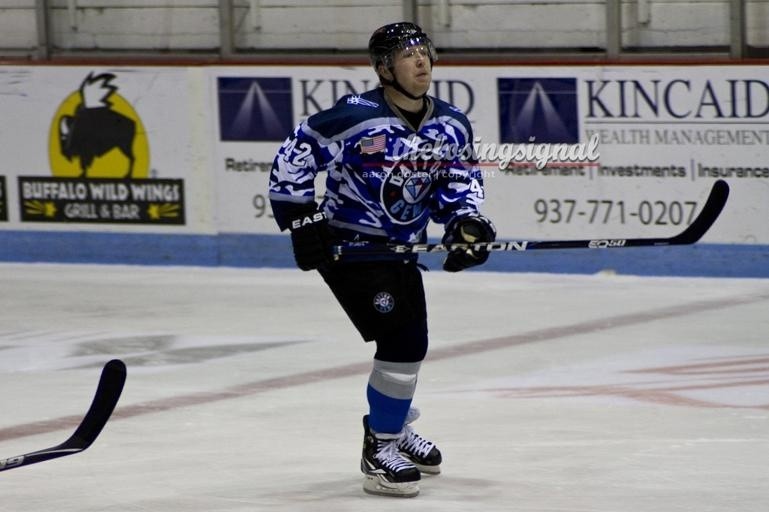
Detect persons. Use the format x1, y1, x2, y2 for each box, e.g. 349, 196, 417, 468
266, 21, 497, 498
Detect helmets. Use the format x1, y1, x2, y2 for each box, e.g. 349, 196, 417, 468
366, 20, 441, 71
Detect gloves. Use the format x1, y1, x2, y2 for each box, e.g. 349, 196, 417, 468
442, 216, 497, 274
286, 206, 349, 273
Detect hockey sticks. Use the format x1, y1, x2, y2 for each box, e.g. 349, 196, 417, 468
336, 178, 729, 259
0, 360, 127, 470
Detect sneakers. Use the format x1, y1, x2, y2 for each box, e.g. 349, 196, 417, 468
399, 405, 443, 469
357, 412, 423, 486
